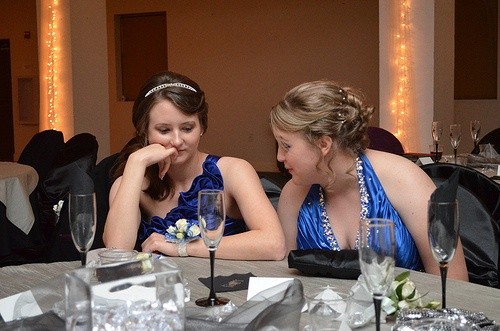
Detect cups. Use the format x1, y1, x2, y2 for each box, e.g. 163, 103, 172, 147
428, 145, 444, 161
304, 286, 354, 331
98, 247, 139, 265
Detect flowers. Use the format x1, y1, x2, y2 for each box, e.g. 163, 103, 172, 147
163, 219, 206, 243
346, 264, 442, 329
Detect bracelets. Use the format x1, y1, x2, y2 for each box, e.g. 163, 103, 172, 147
166, 218, 201, 256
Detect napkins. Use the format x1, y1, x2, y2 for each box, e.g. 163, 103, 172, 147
247, 277, 295, 305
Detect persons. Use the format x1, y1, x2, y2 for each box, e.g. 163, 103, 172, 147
271, 80, 469, 282
101, 72, 287, 260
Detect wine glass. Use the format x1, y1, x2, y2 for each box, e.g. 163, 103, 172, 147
431, 121, 441, 162
358, 219, 396, 331
195, 189, 230, 307
427, 201, 461, 309
470, 120, 481, 149
68, 190, 97, 267
449, 124, 462, 164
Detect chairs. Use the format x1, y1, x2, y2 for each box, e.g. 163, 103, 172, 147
0, 127, 500, 291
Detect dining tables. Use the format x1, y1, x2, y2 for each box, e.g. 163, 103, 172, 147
0, 252, 500, 331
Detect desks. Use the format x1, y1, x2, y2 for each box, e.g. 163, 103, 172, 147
0, 161, 39, 268
